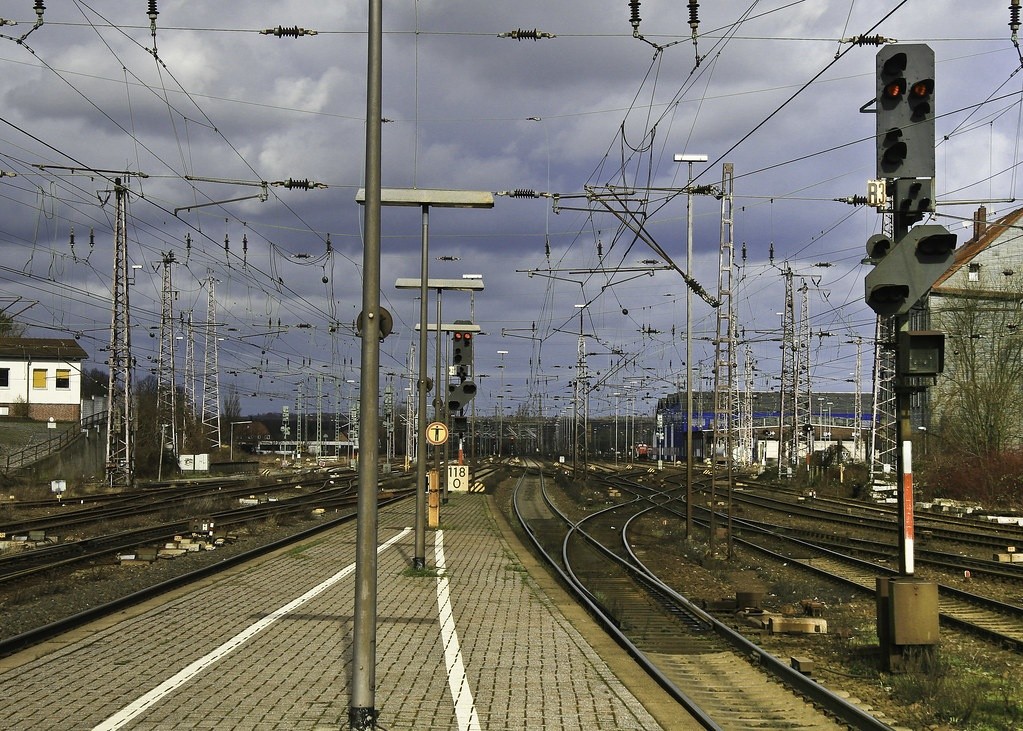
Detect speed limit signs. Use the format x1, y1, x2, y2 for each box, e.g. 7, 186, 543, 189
447, 466, 469, 492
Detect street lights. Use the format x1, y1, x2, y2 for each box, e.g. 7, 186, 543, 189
231, 421, 252, 461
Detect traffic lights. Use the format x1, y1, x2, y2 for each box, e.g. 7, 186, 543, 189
452, 320, 473, 365
457, 366, 471, 378
899, 329, 947, 376
865, 225, 958, 316
454, 416, 468, 433
874, 42, 937, 177
447, 380, 477, 411
